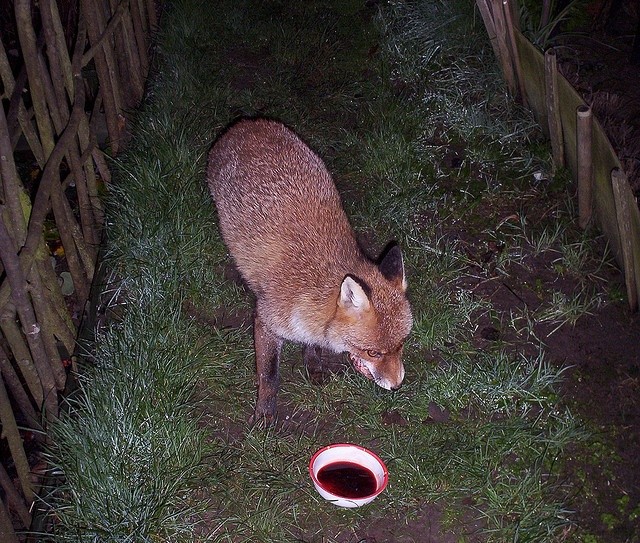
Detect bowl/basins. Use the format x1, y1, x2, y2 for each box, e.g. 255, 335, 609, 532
309, 443, 388, 509
